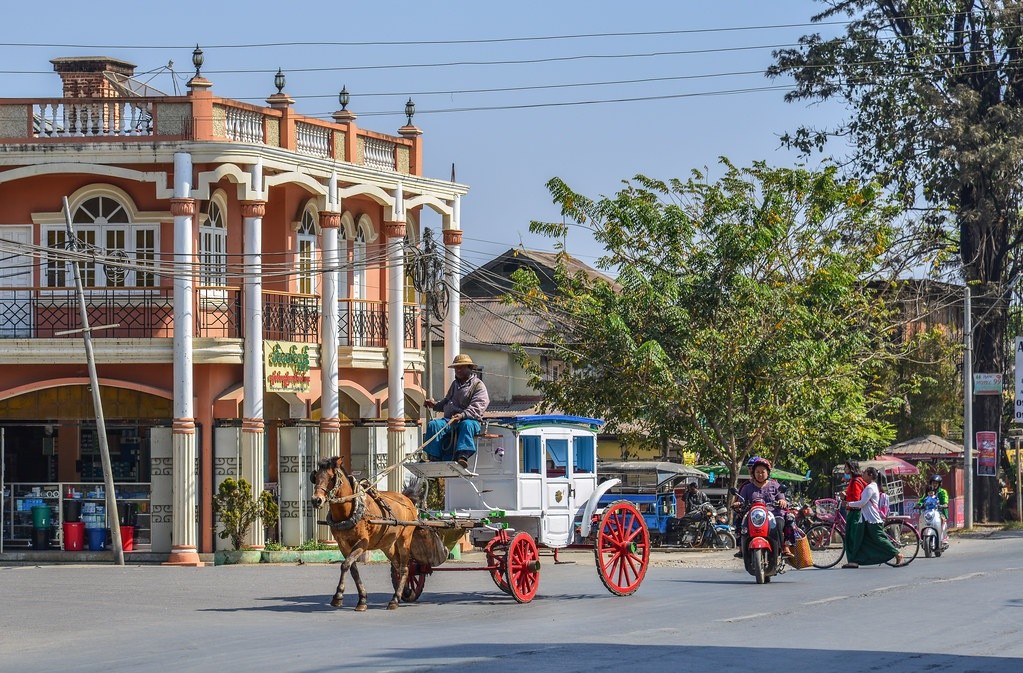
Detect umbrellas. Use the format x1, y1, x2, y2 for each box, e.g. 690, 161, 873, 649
872, 455, 919, 476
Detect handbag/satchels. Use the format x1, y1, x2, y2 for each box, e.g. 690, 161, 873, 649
783, 512, 813, 570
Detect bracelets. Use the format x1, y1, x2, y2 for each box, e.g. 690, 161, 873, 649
846, 502, 848, 506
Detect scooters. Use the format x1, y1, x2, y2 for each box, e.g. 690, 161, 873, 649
912, 499, 950, 559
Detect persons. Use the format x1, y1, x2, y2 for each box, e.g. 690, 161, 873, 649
495, 448, 504, 461
839, 459, 903, 569
423, 354, 489, 469
681, 483, 707, 547
732, 456, 796, 558
915, 474, 948, 544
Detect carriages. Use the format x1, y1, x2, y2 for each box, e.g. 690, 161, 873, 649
308, 412, 651, 613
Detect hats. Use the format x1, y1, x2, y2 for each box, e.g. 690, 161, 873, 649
447, 353, 478, 368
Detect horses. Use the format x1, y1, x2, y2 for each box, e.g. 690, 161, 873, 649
310, 456, 420, 611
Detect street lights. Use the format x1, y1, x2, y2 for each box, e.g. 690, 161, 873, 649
964, 273, 1023, 531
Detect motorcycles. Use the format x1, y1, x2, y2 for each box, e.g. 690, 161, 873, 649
593, 460, 833, 553
728, 483, 790, 584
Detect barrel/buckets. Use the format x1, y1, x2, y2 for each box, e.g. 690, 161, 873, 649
120, 526, 135, 551
62, 500, 81, 521
117, 503, 137, 526
87, 528, 107, 551
63, 522, 85, 551
32, 506, 51, 530
31, 530, 50, 550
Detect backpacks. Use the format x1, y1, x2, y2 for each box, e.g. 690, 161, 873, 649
869, 492, 890, 517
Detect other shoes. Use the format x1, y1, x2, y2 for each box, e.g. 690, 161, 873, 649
782, 550, 794, 557
734, 552, 742, 557
458, 457, 468, 468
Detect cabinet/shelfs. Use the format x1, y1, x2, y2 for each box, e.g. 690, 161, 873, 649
3, 481, 152, 548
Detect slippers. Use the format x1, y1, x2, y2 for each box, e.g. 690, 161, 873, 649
842, 564, 858, 568
893, 562, 910, 567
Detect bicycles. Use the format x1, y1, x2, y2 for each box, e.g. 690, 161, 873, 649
805, 493, 921, 569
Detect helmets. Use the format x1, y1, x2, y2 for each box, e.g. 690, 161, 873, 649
929, 475, 943, 482
752, 458, 771, 475
746, 456, 759, 465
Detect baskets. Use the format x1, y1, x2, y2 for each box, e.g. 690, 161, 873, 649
815, 498, 838, 520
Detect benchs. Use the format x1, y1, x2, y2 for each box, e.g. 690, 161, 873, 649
530, 469, 589, 480
402, 433, 504, 479
